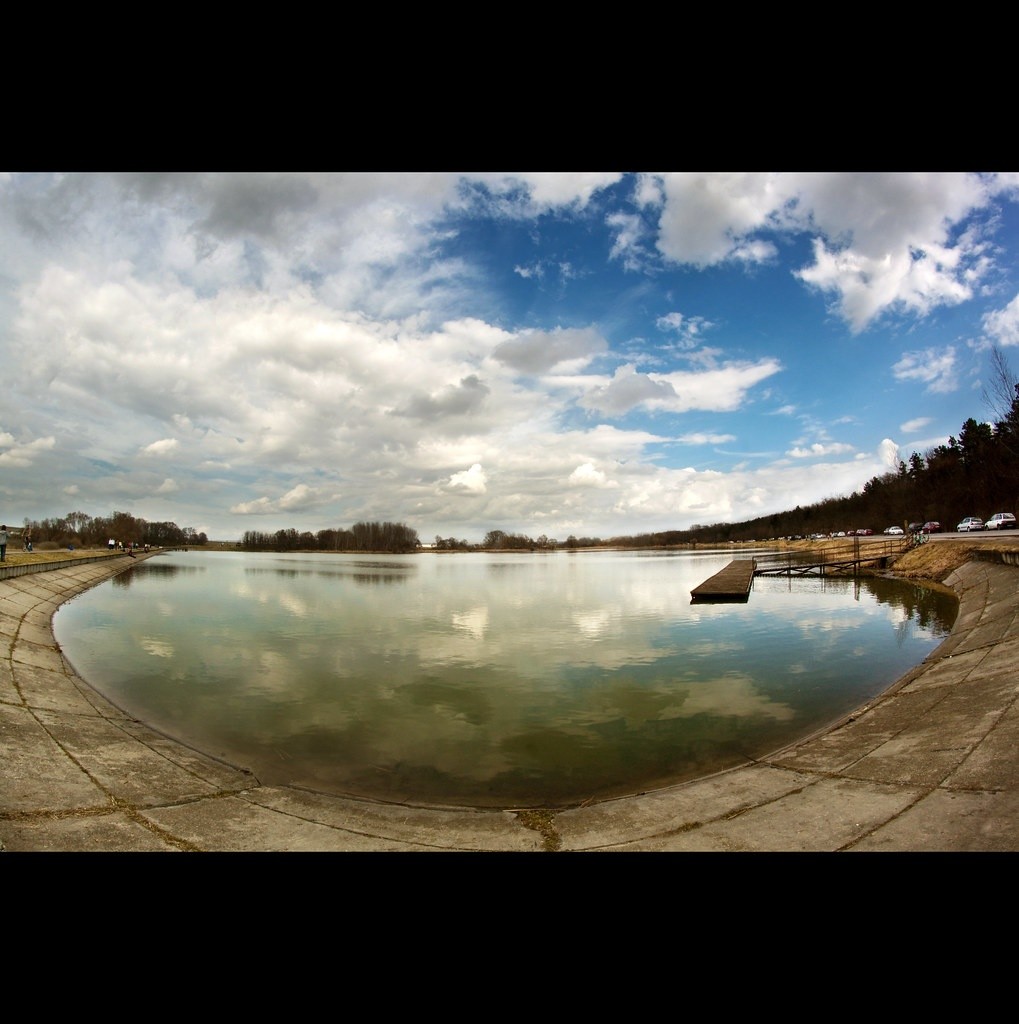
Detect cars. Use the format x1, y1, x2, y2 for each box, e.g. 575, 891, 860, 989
847, 531, 856, 537
984, 513, 1016, 531
957, 517, 984, 532
889, 526, 904, 535
884, 528, 890, 536
837, 532, 846, 537
864, 529, 873, 536
920, 522, 942, 534
908, 522, 924, 533
856, 529, 866, 536
728, 532, 837, 544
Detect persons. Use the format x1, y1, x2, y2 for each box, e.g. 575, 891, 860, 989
914, 529, 923, 548
0, 525, 11, 562
21, 525, 34, 552
108, 537, 139, 556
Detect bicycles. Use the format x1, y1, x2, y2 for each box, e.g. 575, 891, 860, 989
910, 526, 931, 549
21, 535, 32, 552
